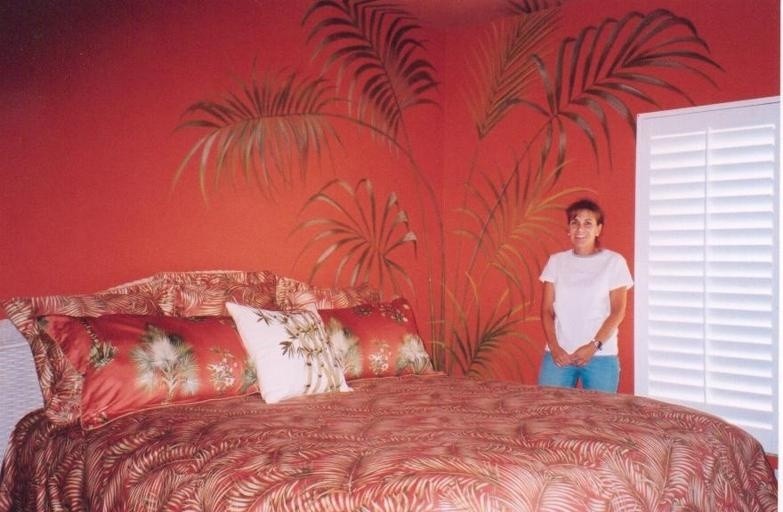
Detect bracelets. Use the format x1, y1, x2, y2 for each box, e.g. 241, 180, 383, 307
591, 339, 602, 351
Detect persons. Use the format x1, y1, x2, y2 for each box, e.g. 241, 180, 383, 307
529, 198, 634, 399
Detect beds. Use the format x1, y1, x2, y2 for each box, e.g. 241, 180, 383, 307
0, 316, 777, 512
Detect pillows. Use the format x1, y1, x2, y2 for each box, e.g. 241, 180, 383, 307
273, 274, 382, 309
317, 297, 435, 382
66, 314, 260, 431
226, 300, 355, 406
5, 292, 165, 430
